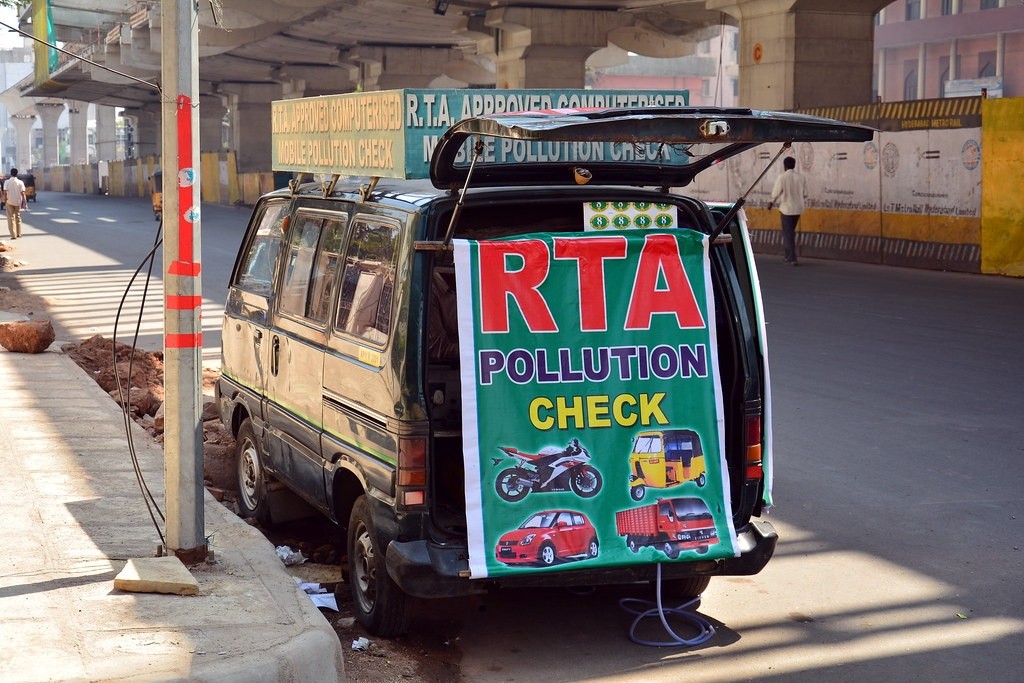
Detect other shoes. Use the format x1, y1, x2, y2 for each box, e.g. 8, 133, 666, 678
18, 234, 23, 238
783, 258, 789, 264
10, 237, 18, 240
791, 261, 799, 266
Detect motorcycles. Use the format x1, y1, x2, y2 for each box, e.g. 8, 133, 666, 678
147, 171, 163, 221
0, 174, 37, 208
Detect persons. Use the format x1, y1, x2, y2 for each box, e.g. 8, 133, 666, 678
0, 168, 27, 239
768, 157, 808, 267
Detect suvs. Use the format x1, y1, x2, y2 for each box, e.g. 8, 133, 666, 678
219, 103, 879, 624
